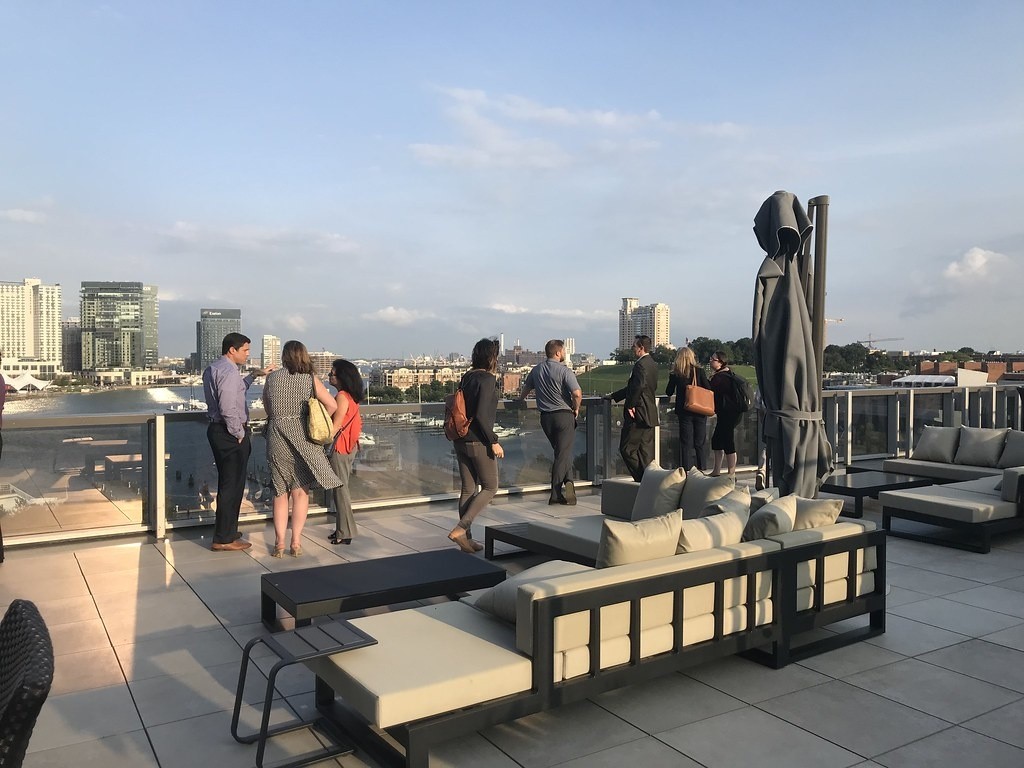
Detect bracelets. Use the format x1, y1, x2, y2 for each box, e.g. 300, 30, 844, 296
250, 373, 256, 379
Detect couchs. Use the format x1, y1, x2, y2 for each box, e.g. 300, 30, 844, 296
230, 477, 888, 768
880, 458, 1024, 554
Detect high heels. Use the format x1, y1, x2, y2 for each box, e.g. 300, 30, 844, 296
271, 543, 286, 559
331, 536, 352, 545
289, 543, 302, 558
327, 530, 336, 539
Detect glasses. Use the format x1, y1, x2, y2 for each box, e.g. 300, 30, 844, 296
710, 356, 721, 362
328, 373, 337, 376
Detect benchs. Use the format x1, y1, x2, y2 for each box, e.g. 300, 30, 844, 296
484, 521, 598, 565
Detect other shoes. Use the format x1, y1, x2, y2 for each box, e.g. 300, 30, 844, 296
755, 473, 765, 491
708, 473, 720, 477
734, 476, 738, 483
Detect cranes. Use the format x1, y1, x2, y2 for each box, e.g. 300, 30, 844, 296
823, 318, 844, 355
857, 334, 905, 349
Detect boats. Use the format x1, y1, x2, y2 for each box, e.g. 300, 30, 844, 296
167, 396, 268, 436
366, 412, 533, 437
359, 433, 377, 446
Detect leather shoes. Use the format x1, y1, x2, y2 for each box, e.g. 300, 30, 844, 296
548, 496, 568, 505
448, 525, 475, 553
461, 538, 483, 551
212, 540, 252, 551
564, 481, 577, 505
234, 531, 243, 540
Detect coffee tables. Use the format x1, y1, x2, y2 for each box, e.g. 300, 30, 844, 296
821, 471, 933, 518
259, 548, 507, 629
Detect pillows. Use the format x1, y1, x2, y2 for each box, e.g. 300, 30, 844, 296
794, 495, 844, 531
910, 424, 960, 463
678, 509, 750, 556
596, 508, 684, 570
679, 465, 735, 519
748, 488, 780, 515
997, 428, 1024, 468
476, 559, 597, 623
955, 424, 1012, 467
631, 458, 686, 521
698, 485, 751, 531
740, 494, 798, 540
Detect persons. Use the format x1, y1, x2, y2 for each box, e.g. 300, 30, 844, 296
512, 339, 583, 504
202, 480, 208, 494
188, 474, 194, 487
262, 340, 337, 558
324, 359, 364, 545
601, 335, 661, 482
665, 346, 711, 470
203, 333, 266, 553
447, 338, 505, 554
708, 349, 748, 482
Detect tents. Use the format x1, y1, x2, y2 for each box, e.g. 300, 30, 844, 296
0, 369, 51, 394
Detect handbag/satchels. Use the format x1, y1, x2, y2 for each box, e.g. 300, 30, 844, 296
684, 366, 715, 416
305, 372, 334, 444
323, 437, 337, 458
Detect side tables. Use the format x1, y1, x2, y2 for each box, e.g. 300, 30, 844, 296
229, 619, 378, 768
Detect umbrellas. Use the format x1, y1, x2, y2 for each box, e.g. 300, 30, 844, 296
751, 189, 837, 498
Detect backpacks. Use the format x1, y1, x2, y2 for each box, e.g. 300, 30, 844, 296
720, 369, 755, 412
443, 371, 484, 441
